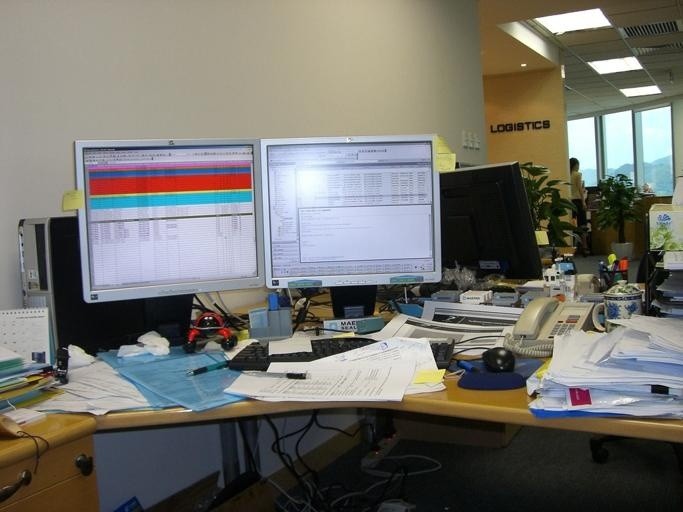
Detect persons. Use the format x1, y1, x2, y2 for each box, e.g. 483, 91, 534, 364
570, 159, 591, 258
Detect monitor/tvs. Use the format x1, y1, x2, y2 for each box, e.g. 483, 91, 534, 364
73, 140, 265, 345
264, 133, 441, 338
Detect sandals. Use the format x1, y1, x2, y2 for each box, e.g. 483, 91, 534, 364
582, 248, 591, 257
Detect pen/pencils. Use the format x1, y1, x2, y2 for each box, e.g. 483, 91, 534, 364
185, 359, 232, 378
598, 260, 619, 287
457, 359, 480, 373
244, 370, 313, 380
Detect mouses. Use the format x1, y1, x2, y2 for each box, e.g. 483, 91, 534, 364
481, 346, 514, 373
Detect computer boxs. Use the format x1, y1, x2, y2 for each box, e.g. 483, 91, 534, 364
18, 218, 194, 369
440, 161, 542, 279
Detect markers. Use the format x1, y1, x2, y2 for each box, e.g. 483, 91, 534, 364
608, 253, 628, 281
267, 292, 280, 313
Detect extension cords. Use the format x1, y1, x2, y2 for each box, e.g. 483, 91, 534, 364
362, 432, 400, 468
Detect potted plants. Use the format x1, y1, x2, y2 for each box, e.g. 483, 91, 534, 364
586, 174, 644, 259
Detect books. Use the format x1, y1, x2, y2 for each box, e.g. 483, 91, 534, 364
651, 274, 683, 318
650, 247, 682, 271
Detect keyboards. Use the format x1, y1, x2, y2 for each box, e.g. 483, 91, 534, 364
228, 337, 454, 368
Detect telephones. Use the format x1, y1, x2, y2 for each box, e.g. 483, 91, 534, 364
501, 298, 594, 358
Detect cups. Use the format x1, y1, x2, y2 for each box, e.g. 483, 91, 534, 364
591, 280, 644, 334
